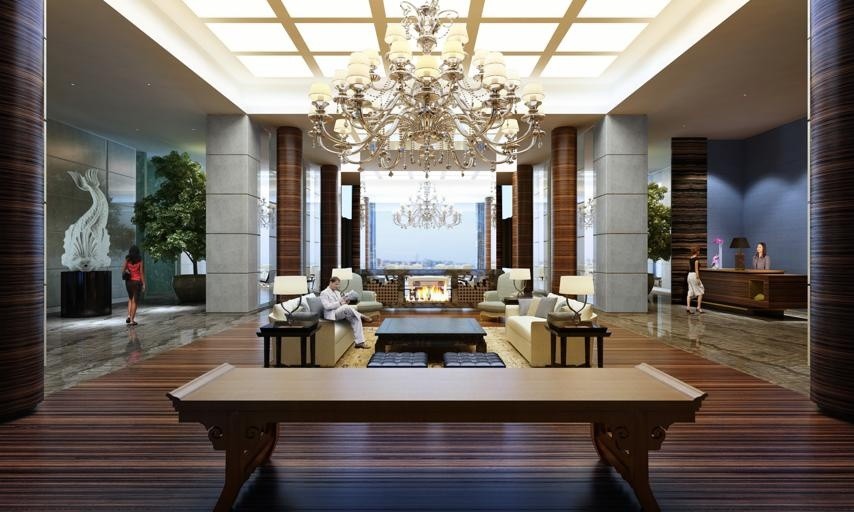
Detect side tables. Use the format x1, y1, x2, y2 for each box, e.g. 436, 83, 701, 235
256, 321, 321, 368
544, 325, 611, 368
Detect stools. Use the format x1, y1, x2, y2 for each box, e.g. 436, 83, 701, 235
443, 351, 506, 368
367, 351, 428, 368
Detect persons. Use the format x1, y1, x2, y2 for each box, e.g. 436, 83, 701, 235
753, 241, 771, 269
120, 245, 146, 325
320, 276, 373, 349
686, 245, 705, 315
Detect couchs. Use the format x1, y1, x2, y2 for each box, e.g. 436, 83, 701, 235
505, 292, 598, 367
268, 293, 358, 367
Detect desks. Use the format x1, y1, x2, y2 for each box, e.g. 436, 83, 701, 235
501, 297, 519, 305
165, 363, 708, 510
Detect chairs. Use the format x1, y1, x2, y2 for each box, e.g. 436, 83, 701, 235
339, 273, 383, 320
477, 273, 525, 322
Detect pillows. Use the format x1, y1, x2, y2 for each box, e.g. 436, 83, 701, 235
517, 297, 571, 318
283, 311, 320, 321
305, 297, 324, 318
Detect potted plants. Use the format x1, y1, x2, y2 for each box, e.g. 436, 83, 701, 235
130, 150, 206, 305
648, 181, 673, 305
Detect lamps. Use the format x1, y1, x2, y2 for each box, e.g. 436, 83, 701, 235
729, 237, 750, 270
391, 179, 462, 231
273, 275, 308, 324
558, 275, 595, 326
332, 268, 352, 297
509, 268, 531, 297
306, 1, 546, 178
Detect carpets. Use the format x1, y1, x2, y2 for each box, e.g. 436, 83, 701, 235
335, 326, 531, 368
